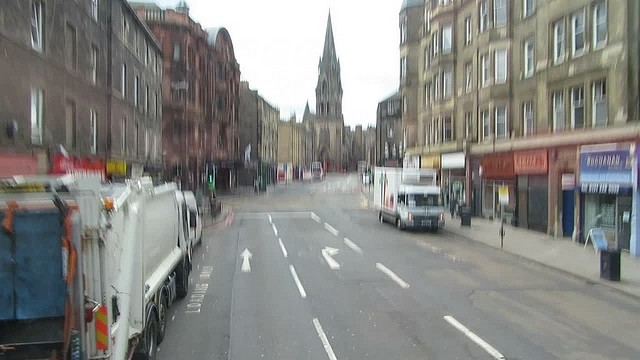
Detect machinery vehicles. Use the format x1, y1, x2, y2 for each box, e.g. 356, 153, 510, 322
0, 172, 201, 359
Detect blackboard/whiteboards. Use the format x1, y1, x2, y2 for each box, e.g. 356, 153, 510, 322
590, 229, 608, 252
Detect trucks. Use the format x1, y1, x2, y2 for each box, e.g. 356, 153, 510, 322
374, 167, 445, 232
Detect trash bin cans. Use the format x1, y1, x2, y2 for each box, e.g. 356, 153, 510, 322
459, 212, 471, 226
601, 249, 620, 281
